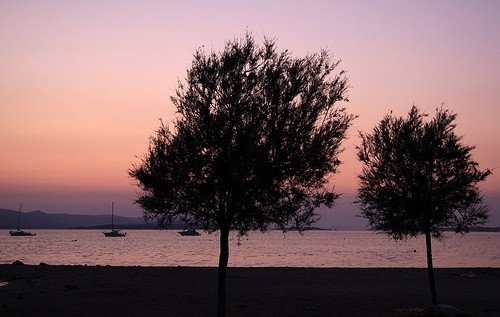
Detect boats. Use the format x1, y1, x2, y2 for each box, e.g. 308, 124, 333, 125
177, 225, 199, 236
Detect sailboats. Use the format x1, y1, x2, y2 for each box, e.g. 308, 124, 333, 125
9, 202, 37, 237
103, 202, 128, 236
